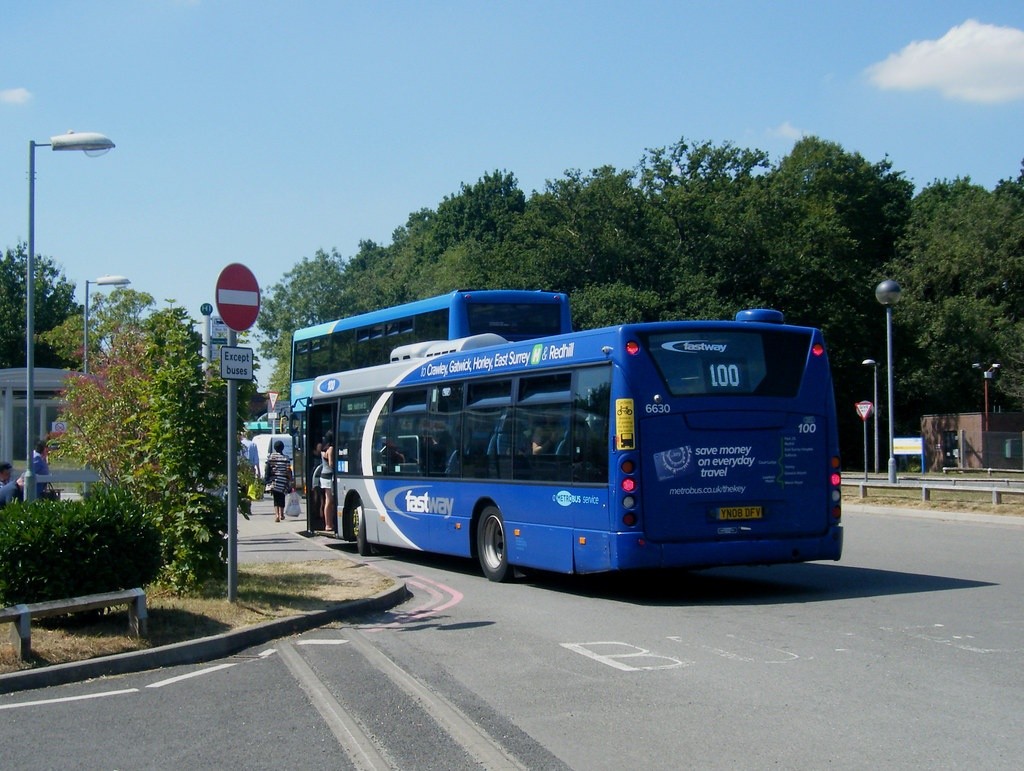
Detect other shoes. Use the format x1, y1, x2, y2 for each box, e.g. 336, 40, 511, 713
275, 518, 280, 522
280, 516, 285, 520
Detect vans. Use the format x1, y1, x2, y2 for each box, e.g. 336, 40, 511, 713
252, 433, 306, 490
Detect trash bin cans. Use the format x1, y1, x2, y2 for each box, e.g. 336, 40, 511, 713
44, 488, 64, 501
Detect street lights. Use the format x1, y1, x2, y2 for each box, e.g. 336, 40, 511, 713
23, 132, 116, 503
972, 361, 1001, 432
861, 359, 878, 474
83, 276, 131, 504
874, 279, 902, 484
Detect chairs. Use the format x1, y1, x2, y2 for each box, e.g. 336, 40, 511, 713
446, 424, 583, 480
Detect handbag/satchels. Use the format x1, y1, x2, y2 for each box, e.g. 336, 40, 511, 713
246, 477, 265, 501
41, 483, 58, 501
265, 481, 275, 491
283, 488, 301, 517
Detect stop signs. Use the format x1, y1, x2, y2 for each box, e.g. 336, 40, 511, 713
216, 262, 260, 333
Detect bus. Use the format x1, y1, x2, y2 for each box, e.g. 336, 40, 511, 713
289, 308, 844, 584
278, 288, 574, 499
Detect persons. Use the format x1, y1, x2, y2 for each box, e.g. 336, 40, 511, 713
264, 440, 294, 522
311, 405, 564, 531
240, 431, 260, 515
0, 440, 50, 511
